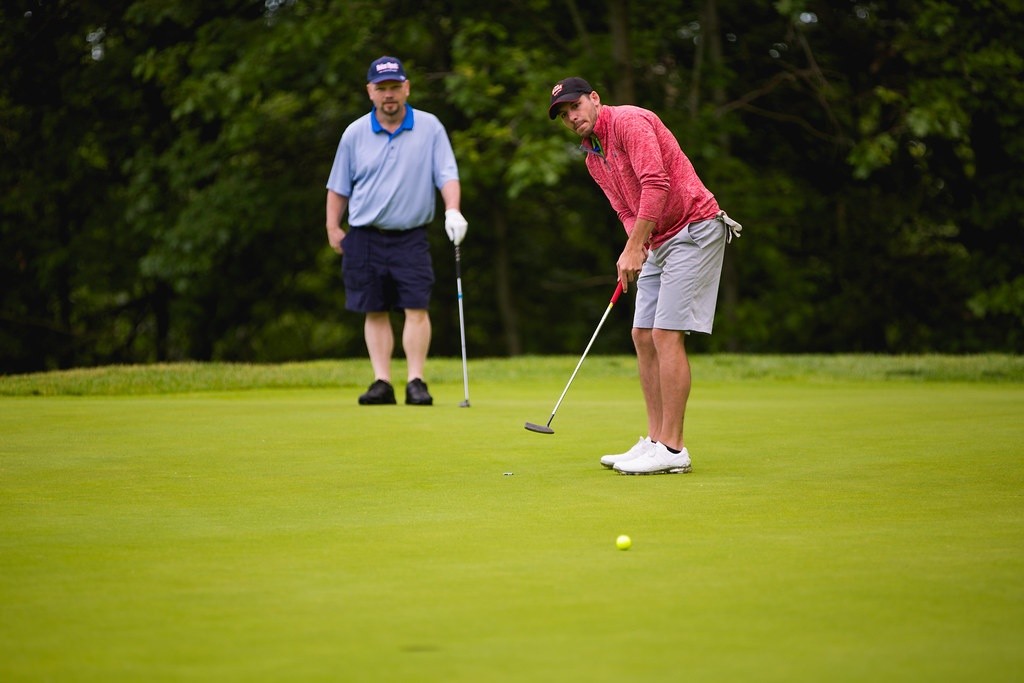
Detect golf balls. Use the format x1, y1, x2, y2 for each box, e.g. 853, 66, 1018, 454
616, 535, 632, 551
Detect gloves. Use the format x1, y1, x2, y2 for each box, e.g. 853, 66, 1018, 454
445, 208, 469, 246
717, 212, 742, 244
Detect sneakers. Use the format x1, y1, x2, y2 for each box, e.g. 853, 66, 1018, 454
613, 442, 692, 476
601, 437, 656, 470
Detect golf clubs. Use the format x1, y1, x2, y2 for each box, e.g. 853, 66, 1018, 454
524, 243, 650, 434
455, 245, 470, 407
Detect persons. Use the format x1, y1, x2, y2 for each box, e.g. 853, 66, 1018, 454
548, 77, 726, 475
326, 55, 468, 405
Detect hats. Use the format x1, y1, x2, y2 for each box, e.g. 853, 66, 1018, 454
367, 56, 406, 84
548, 78, 593, 121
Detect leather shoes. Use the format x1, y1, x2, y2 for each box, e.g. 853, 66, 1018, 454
406, 379, 432, 405
358, 381, 397, 405
